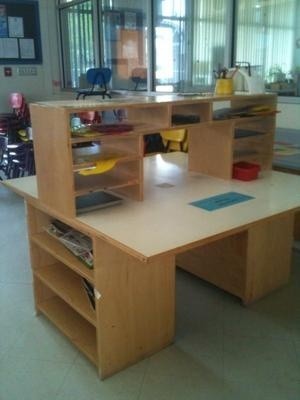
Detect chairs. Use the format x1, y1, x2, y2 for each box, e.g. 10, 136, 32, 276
129, 68, 146, 91
76, 68, 117, 117
0, 93, 101, 179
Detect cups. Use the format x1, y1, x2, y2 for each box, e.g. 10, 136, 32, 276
248, 79, 266, 94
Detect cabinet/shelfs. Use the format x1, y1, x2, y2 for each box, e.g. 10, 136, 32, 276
28, 202, 175, 381
27, 91, 278, 216
